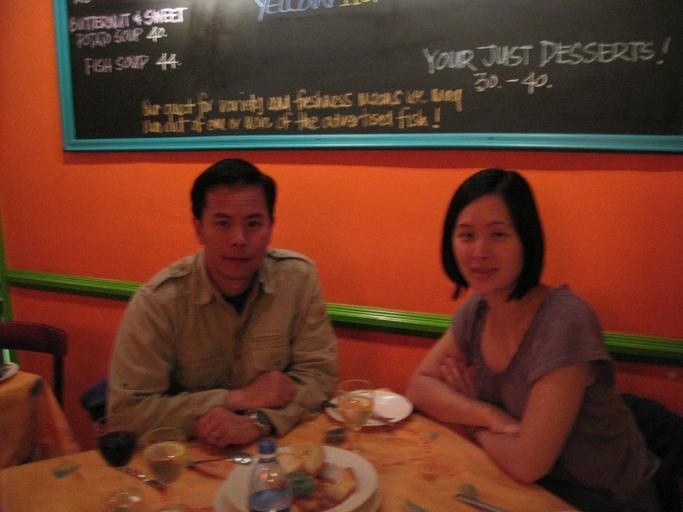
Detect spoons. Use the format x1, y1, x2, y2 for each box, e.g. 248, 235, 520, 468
185, 454, 251, 468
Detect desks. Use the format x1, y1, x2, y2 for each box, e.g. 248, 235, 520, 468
0, 371, 582, 512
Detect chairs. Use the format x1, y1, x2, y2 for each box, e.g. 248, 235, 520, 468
0, 320, 70, 412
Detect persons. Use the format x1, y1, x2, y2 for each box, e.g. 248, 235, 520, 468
100, 156, 341, 452
405, 166, 672, 511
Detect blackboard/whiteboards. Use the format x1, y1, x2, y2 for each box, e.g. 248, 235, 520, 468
54, 0, 683, 154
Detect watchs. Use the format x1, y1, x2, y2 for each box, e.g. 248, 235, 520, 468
248, 411, 270, 436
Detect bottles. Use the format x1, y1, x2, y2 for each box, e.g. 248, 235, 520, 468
248, 438, 290, 512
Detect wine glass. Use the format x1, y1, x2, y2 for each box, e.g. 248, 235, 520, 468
96, 415, 186, 510
335, 381, 373, 455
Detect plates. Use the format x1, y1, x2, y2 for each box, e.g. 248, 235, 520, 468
216, 445, 378, 512
324, 389, 415, 427
